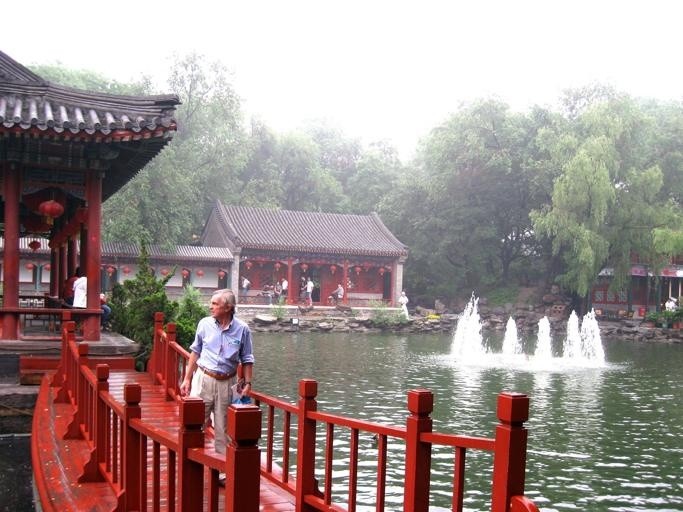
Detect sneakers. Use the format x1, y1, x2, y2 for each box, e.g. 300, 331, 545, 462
219, 473, 227, 485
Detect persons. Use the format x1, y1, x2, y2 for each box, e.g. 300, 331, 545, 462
62, 266, 112, 331
240, 274, 252, 304
301, 276, 315, 307
398, 291, 409, 320
664, 298, 675, 312
257, 277, 288, 306
72, 273, 87, 308
179, 287, 255, 487
300, 276, 306, 305
331, 282, 344, 304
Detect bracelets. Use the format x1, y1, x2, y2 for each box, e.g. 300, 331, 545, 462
244, 381, 251, 386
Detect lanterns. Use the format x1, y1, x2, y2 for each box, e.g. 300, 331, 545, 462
28, 241, 41, 252
245, 261, 386, 276
107, 266, 226, 280
24, 263, 34, 272
44, 263, 51, 272
23, 193, 65, 237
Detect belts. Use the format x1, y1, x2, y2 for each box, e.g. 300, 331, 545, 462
199, 366, 238, 380
